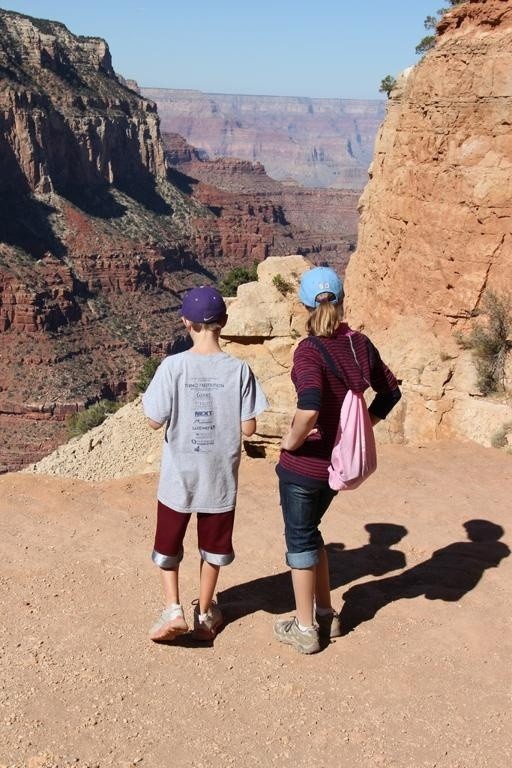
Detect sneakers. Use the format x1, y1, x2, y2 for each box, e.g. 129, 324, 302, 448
149, 608, 189, 641
273, 616, 320, 654
312, 606, 342, 638
191, 599, 223, 641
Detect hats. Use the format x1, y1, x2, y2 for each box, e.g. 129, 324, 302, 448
299, 266, 343, 308
177, 285, 226, 323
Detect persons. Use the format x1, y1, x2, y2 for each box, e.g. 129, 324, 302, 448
272, 265, 402, 653
142, 284, 272, 644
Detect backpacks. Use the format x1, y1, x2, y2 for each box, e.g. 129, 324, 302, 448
308, 335, 377, 491
